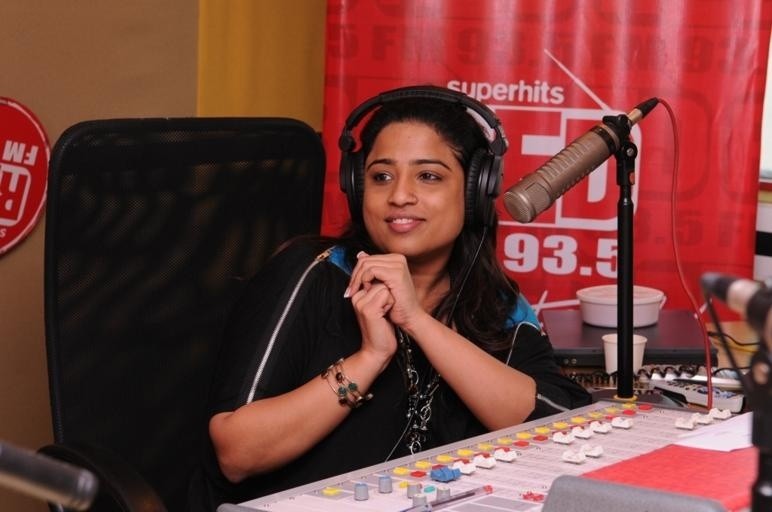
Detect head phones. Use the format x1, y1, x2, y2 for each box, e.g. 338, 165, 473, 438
338, 85, 509, 232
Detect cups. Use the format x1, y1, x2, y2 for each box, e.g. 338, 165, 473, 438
601, 332, 649, 378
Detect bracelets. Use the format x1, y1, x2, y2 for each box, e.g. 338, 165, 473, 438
320, 357, 373, 411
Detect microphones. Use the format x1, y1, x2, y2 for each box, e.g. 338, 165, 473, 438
701, 270, 772, 347
501, 96, 658, 224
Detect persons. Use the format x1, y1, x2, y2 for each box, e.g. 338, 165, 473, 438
202, 96, 593, 508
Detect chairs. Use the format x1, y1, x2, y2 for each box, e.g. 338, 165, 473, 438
37, 115, 328, 509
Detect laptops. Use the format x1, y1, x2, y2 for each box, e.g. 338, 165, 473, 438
542, 309, 719, 368
